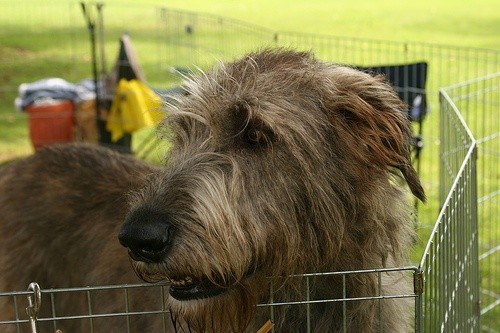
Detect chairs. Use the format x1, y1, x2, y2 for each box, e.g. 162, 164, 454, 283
343, 60, 426, 204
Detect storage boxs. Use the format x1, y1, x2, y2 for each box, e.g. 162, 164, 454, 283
26, 98, 76, 150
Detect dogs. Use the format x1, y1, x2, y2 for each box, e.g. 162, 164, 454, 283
0, 42, 430, 333
